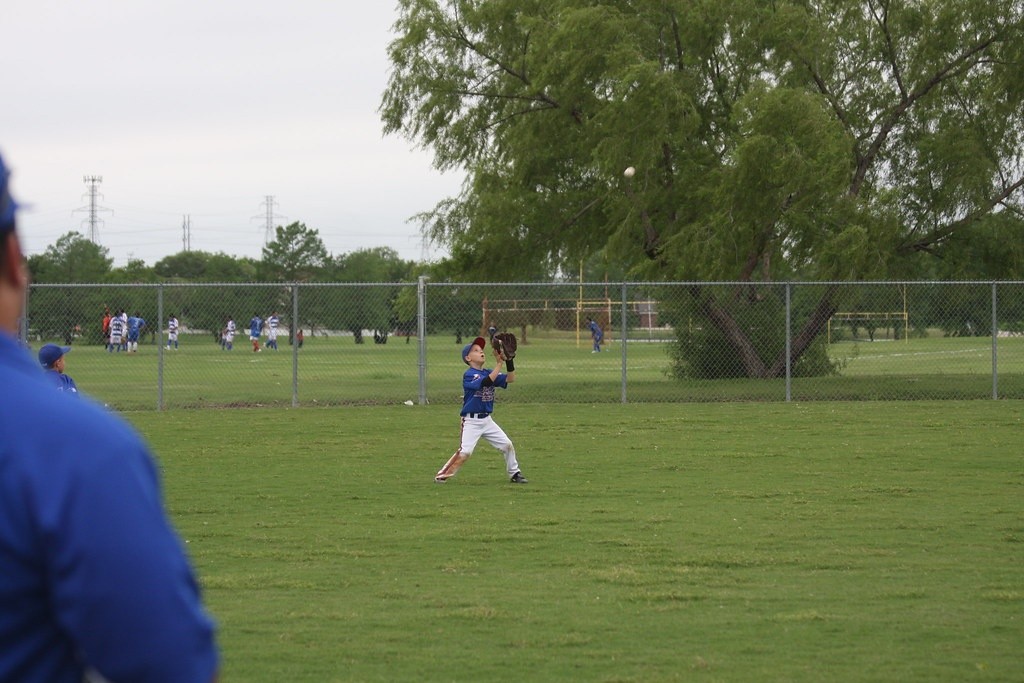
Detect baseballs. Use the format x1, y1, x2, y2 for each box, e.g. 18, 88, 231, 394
624, 166, 636, 178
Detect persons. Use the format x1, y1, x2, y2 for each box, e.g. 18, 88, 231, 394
433, 333, 529, 484
163, 312, 179, 351
488, 320, 498, 343
297, 328, 303, 348
101, 301, 147, 354
221, 315, 236, 352
0, 152, 221, 683
39, 344, 77, 395
586, 317, 604, 353
263, 311, 280, 352
246, 311, 265, 353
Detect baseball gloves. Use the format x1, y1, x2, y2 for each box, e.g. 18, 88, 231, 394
491, 331, 517, 362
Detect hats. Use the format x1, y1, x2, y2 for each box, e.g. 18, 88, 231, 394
0, 157, 17, 232
462, 337, 485, 365
37, 344, 71, 368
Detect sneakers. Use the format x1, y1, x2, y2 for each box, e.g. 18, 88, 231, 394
511, 472, 527, 483
435, 476, 445, 483
